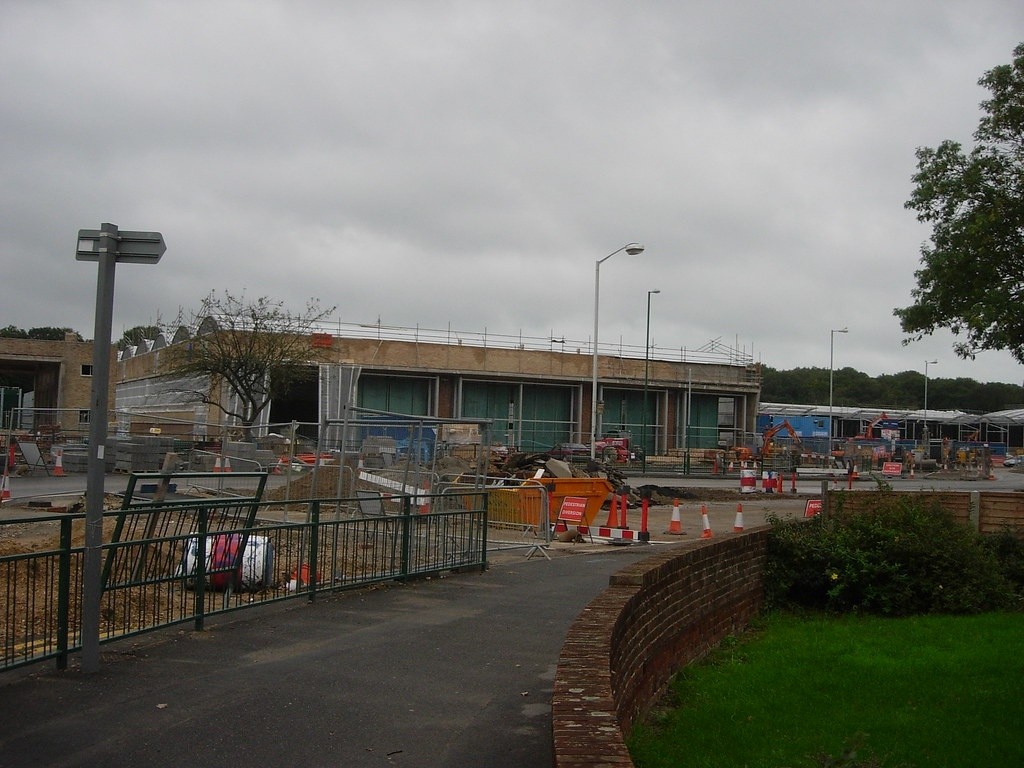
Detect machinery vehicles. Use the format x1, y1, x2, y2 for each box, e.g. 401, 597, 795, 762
831, 412, 896, 463
697, 419, 802, 463
586, 430, 644, 463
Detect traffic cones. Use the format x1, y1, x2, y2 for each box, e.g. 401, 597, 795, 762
224, 456, 233, 472
286, 564, 320, 591
852, 464, 860, 477
701, 506, 713, 539
729, 460, 759, 473
214, 455, 221, 472
417, 479, 431, 523
49, 450, 68, 476
0, 467, 10, 499
663, 498, 687, 535
271, 456, 283, 474
734, 503, 743, 532
607, 496, 618, 528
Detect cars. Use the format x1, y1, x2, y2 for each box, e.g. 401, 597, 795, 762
493, 447, 508, 456
544, 442, 591, 462
1003, 455, 1024, 466
505, 440, 554, 453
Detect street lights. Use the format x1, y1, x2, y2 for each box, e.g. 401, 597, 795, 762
923, 360, 938, 457
591, 241, 644, 459
642, 290, 660, 471
828, 329, 848, 465
